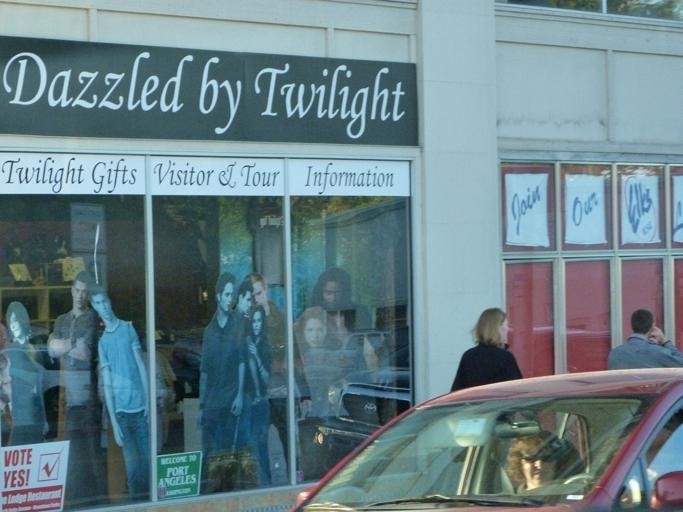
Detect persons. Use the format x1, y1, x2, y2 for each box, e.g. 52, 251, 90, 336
0, 323, 12, 447
87, 284, 156, 503
602, 310, 682, 370
41, 269, 111, 511
0, 298, 51, 446
446, 306, 524, 394
500, 428, 570, 494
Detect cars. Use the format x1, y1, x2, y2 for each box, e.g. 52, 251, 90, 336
279, 367, 683, 512
1, 344, 96, 413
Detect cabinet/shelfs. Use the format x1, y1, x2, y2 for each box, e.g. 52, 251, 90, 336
0, 283, 89, 444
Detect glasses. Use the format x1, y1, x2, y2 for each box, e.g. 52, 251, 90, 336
522, 455, 556, 463
